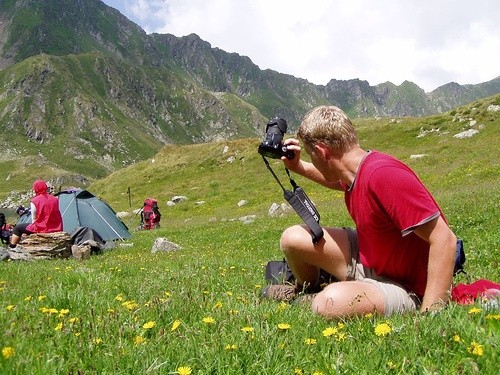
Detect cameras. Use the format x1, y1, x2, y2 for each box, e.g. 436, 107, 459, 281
257, 117, 294, 160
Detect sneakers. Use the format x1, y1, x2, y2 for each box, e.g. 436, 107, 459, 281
4, 244, 19, 253
259, 283, 317, 312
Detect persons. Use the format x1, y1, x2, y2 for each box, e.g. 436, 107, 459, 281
16, 205, 26, 216
9, 180, 63, 249
259, 106, 458, 318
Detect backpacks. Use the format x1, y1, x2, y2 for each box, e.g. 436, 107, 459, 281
140, 199, 161, 229
0, 212, 7, 230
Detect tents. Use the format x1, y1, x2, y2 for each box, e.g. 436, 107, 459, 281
13, 189, 133, 242
